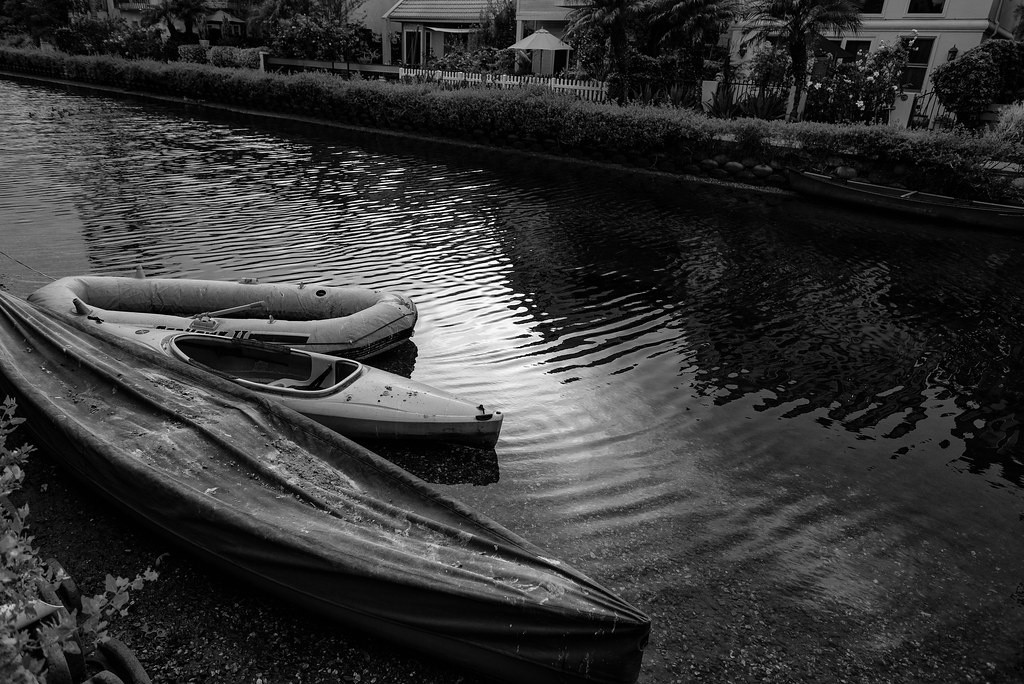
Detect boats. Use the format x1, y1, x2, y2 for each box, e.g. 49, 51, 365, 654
26, 263, 419, 364
782, 164, 1024, 234
82, 314, 505, 452
1, 289, 653, 684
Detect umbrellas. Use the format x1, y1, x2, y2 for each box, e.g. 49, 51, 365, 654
202, 9, 246, 25
506, 25, 574, 80
